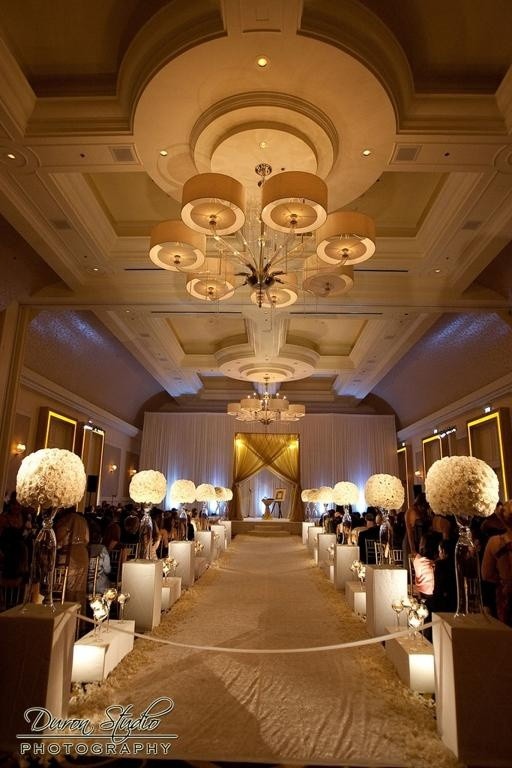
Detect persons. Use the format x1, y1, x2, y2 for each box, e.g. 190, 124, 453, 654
1, 490, 223, 632
318, 502, 512, 632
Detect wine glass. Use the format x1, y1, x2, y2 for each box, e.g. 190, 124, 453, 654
161, 555, 178, 586
350, 560, 366, 591
88, 588, 131, 642
392, 595, 429, 649
326, 542, 335, 562
192, 538, 205, 559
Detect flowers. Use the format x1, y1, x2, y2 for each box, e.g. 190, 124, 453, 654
16, 448, 86, 520
364, 474, 405, 516
301, 481, 359, 506
128, 469, 166, 513
170, 479, 233, 502
424, 456, 500, 527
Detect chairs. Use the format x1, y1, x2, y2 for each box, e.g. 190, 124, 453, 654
0, 527, 188, 612
340, 521, 448, 597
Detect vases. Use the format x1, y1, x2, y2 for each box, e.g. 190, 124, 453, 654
20, 519, 58, 614
322, 506, 332, 533
379, 516, 396, 569
136, 509, 154, 560
178, 507, 188, 542
454, 528, 491, 626
341, 506, 353, 545
199, 503, 209, 531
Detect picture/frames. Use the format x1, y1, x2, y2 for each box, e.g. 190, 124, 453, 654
273, 488, 287, 502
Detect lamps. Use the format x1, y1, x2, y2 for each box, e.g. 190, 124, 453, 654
105, 464, 117, 474
11, 442, 26, 455
148, 163, 376, 310
227, 377, 306, 426
